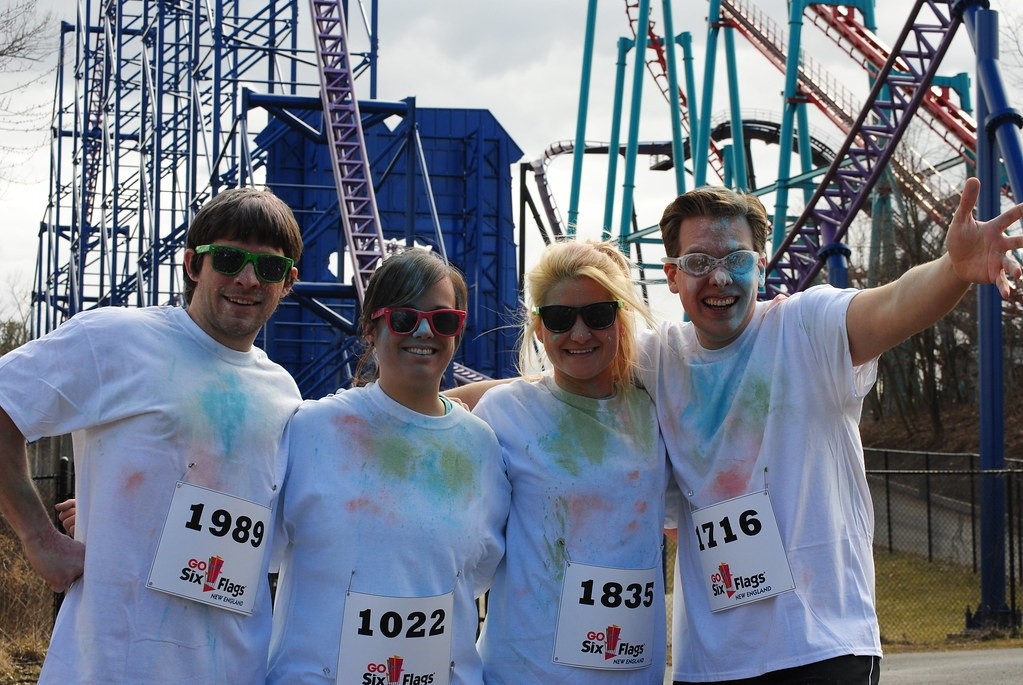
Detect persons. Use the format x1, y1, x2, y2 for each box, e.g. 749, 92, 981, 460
328, 177, 1023, 685
55, 250, 511, 685
470, 239, 679, 685
0, 189, 469, 685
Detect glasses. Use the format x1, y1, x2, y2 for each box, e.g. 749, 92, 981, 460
661, 250, 766, 278
196, 244, 294, 284
370, 306, 467, 337
534, 300, 623, 333
